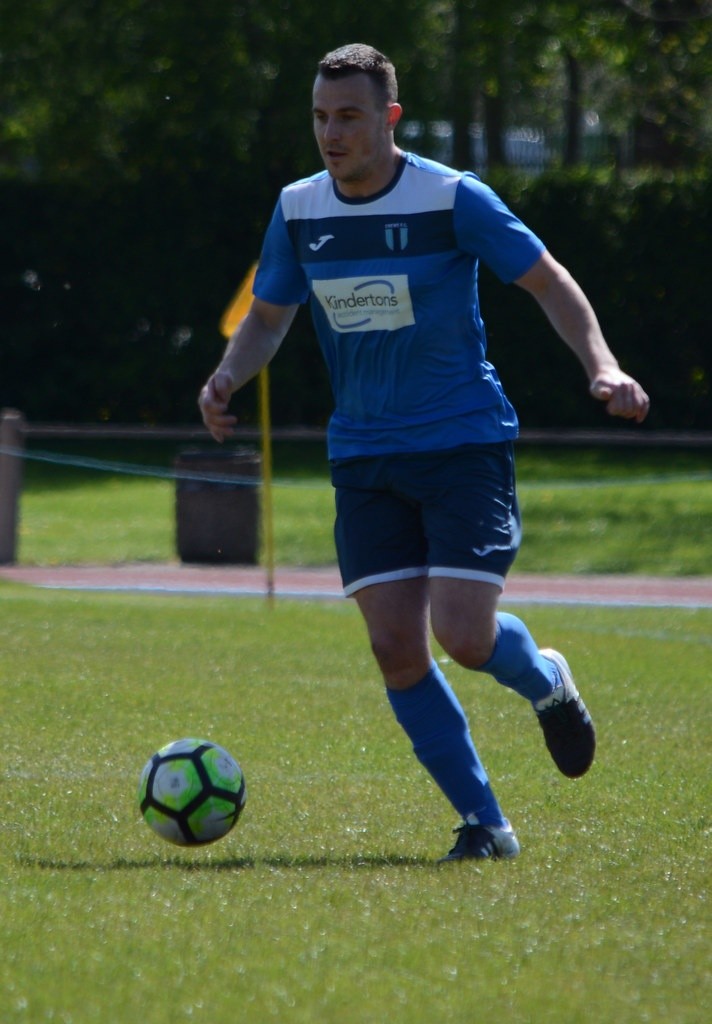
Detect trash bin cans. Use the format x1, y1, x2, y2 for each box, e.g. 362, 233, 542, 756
175, 448, 261, 567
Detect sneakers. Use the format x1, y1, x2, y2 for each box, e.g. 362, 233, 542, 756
537, 646, 596, 780
438, 816, 522, 865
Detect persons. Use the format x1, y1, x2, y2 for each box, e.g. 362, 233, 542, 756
198, 44, 650, 863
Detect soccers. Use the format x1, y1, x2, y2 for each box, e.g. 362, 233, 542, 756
137, 737, 248, 848
